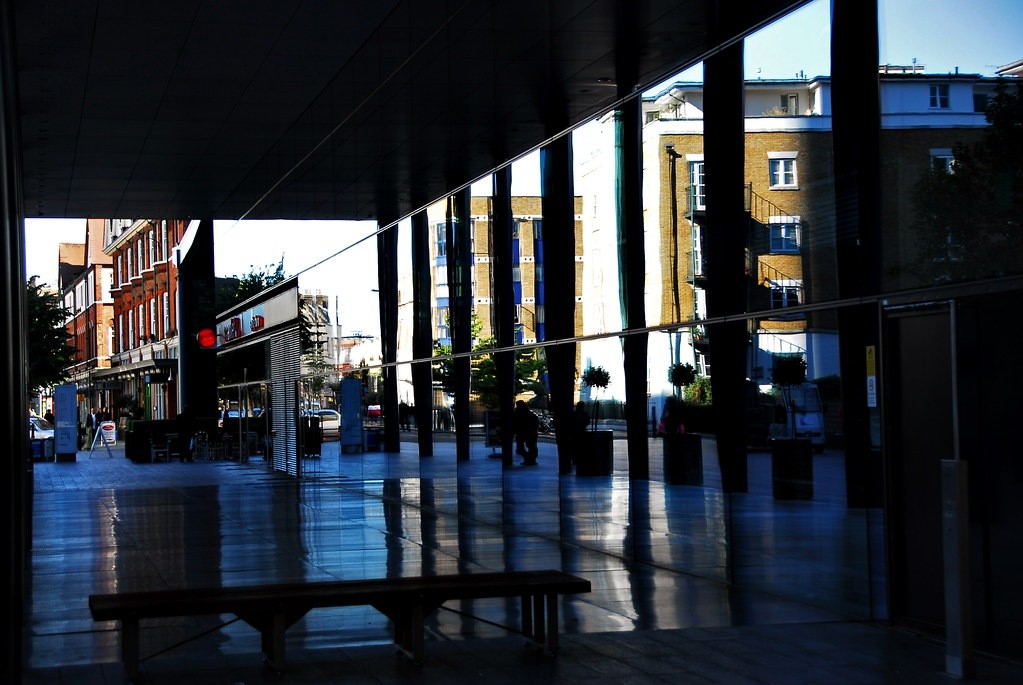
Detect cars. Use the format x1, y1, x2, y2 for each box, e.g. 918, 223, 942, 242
29, 417, 54, 439
307, 409, 341, 440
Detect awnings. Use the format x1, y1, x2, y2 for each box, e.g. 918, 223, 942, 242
91, 359, 178, 382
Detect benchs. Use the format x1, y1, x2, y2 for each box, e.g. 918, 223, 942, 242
89, 570, 591, 685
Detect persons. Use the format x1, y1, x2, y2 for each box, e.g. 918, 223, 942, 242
30, 408, 36, 417
515, 398, 539, 466
575, 401, 590, 433
44, 409, 55, 425
86, 405, 110, 453
659, 410, 686, 434
398, 400, 411, 431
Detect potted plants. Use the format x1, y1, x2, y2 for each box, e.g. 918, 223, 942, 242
575, 366, 614, 477
484, 367, 524, 459
661, 364, 703, 486
768, 354, 813, 500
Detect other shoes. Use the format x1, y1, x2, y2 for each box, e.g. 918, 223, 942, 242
520, 460, 536, 466
407, 427, 410, 431
403, 429, 405, 431
